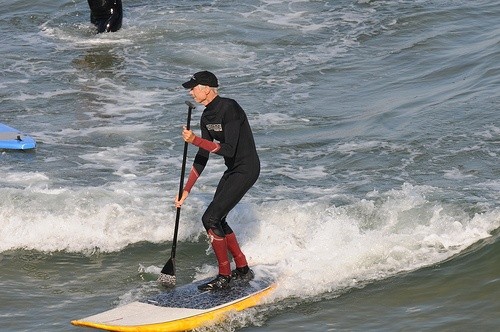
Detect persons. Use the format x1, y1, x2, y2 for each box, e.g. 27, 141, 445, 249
175, 70, 261, 292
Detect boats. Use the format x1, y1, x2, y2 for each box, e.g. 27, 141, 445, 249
69, 256, 304, 332
0, 122, 37, 151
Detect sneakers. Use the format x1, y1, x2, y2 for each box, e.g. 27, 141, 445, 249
182, 71, 218, 88
231, 268, 254, 288
197, 275, 233, 291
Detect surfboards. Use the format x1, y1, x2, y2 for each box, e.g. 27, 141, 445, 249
71, 263, 281, 332
0, 123, 36, 148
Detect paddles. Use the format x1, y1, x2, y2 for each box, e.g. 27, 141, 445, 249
161, 101, 195, 275
